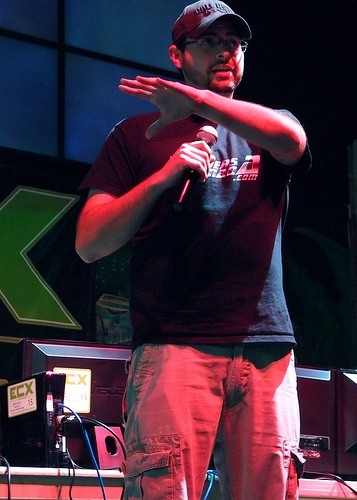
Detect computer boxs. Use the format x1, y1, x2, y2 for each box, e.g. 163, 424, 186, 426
0, 372, 65, 467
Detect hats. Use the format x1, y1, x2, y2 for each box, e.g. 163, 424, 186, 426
171, 0, 252, 41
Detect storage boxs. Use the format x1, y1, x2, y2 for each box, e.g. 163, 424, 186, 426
96, 293, 134, 346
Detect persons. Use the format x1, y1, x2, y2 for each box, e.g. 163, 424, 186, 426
74, 0, 313, 500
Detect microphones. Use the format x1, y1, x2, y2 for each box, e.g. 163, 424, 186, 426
172, 125, 218, 212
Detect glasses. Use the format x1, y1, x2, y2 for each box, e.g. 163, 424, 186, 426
179, 36, 249, 53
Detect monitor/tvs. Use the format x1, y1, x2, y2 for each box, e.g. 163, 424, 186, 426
295, 366, 357, 480
18, 337, 133, 465
